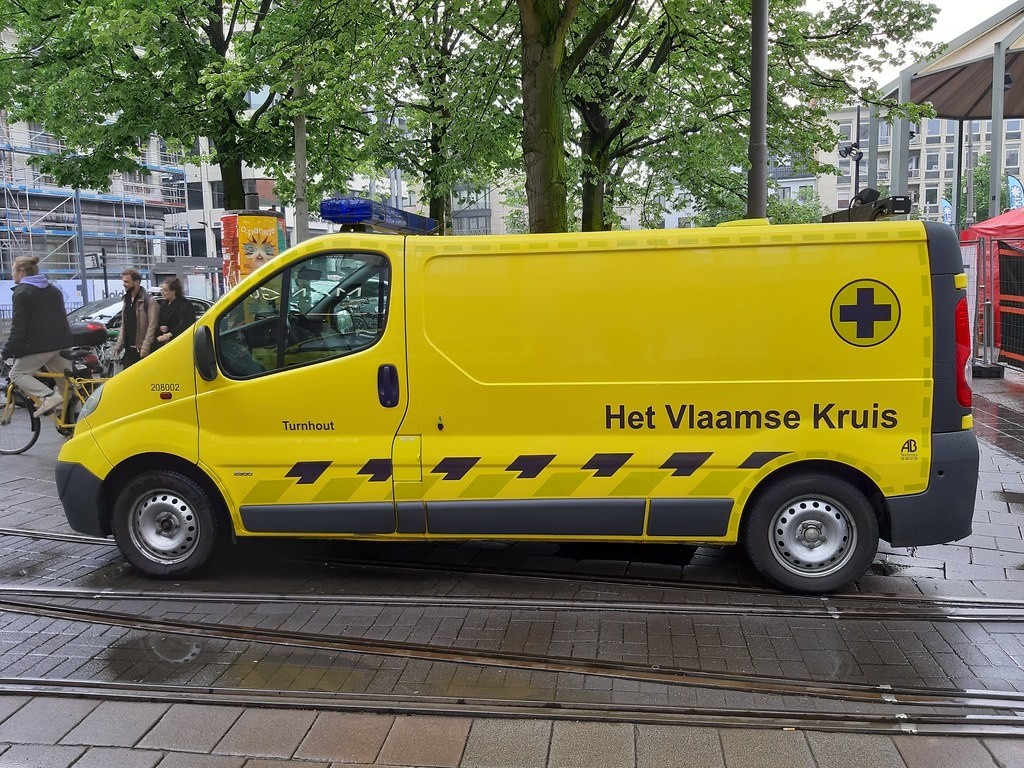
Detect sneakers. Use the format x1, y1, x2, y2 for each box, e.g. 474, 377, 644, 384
33, 392, 64, 418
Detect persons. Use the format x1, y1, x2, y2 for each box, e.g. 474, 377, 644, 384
151, 277, 196, 354
114, 268, 160, 370
0, 256, 81, 420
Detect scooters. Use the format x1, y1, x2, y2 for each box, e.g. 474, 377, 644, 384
70, 321, 109, 379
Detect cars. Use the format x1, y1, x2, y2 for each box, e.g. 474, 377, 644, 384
291, 275, 388, 314
66, 287, 216, 347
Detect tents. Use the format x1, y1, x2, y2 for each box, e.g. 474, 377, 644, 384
961, 206, 1024, 348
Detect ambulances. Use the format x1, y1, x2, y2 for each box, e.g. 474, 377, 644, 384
52, 197, 982, 595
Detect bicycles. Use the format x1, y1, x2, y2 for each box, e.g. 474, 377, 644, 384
346, 301, 377, 331
0, 350, 111, 455
0, 317, 121, 410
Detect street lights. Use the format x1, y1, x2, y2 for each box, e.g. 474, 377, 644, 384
839, 142, 863, 196
